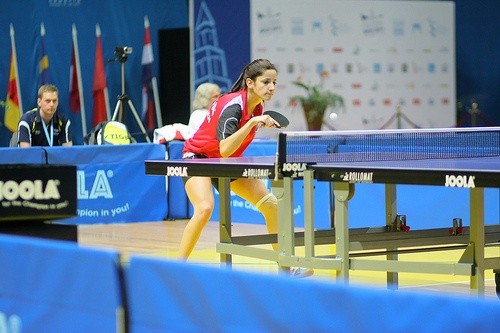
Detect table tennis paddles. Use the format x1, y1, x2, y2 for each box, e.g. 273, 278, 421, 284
258, 111, 289, 129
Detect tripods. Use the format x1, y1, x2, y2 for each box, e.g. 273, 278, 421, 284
108, 57, 151, 143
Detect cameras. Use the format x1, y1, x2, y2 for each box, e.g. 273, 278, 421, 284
114, 46, 132, 58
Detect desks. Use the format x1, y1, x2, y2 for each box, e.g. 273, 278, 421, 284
144, 153, 500, 297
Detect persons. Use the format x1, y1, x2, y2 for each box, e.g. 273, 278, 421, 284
16, 83, 72, 147
173, 56, 314, 277
187, 82, 221, 139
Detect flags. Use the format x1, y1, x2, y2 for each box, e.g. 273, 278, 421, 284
34, 33, 57, 104
4, 45, 23, 132
92, 36, 107, 127
141, 28, 156, 128
67, 42, 80, 114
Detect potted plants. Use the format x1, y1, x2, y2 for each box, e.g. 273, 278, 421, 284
289, 82, 345, 137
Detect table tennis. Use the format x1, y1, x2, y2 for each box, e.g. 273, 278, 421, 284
329, 112, 338, 122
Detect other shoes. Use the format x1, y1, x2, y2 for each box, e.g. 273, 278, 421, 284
290, 267, 312, 279
